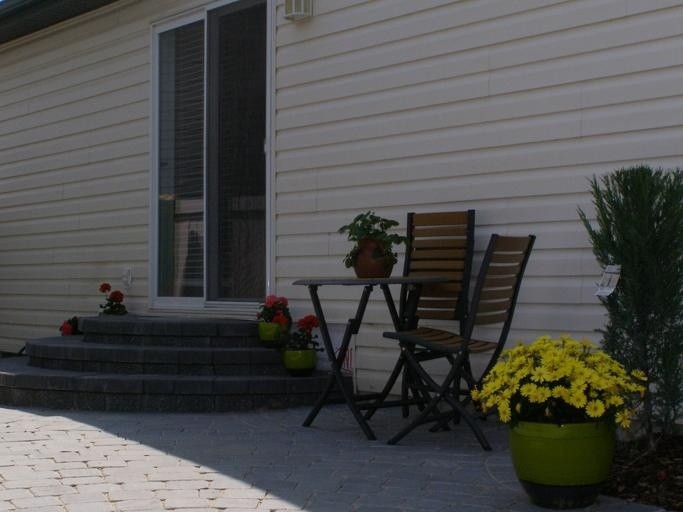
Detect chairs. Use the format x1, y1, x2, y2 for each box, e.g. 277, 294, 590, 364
388, 206, 536, 452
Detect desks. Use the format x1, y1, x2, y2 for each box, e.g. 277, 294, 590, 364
292, 276, 454, 442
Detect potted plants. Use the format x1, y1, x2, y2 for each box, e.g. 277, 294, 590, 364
339, 213, 407, 277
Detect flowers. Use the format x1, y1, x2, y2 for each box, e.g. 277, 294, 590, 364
467, 335, 647, 429
59, 316, 79, 334
99, 282, 126, 314
253, 296, 321, 350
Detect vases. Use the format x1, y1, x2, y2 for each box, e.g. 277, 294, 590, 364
284, 350, 316, 367
509, 419, 616, 505
